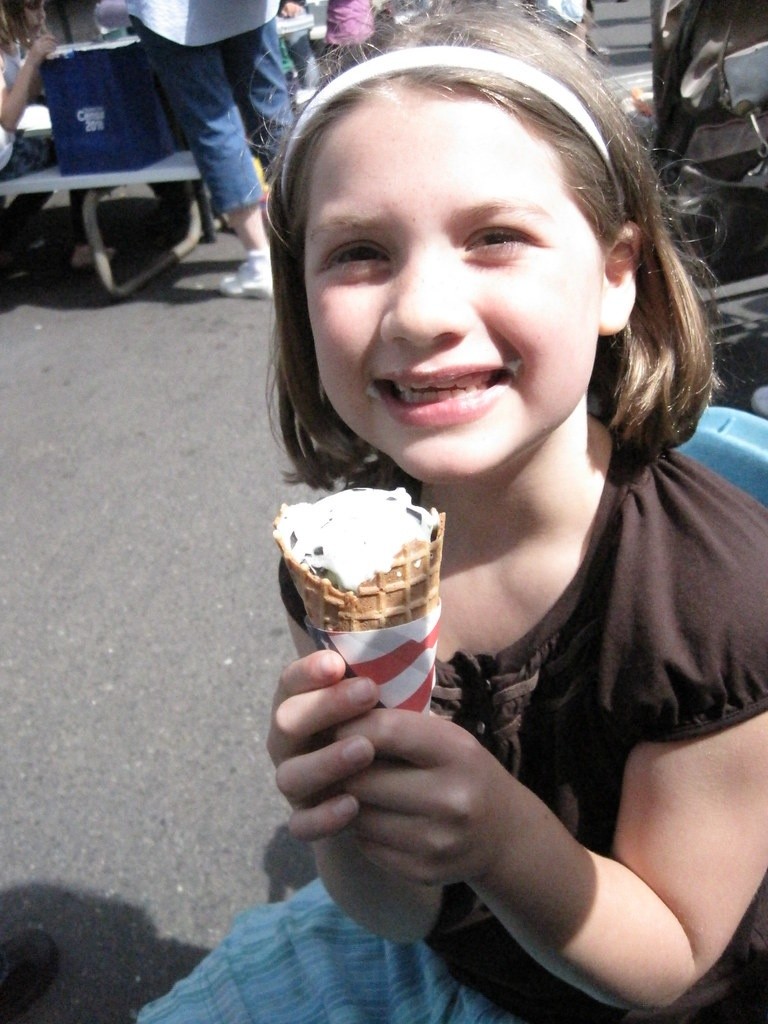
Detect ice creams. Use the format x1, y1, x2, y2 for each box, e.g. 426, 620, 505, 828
274, 486, 446, 631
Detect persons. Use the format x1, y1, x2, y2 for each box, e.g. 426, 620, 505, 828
0, 930, 59, 1023
137, 8, 768, 1024
0, 0, 589, 301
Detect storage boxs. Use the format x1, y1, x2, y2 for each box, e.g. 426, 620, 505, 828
40, 34, 179, 177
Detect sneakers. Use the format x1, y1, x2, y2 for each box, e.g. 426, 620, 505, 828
218, 262, 272, 299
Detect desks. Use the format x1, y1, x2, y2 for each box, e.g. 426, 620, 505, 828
0, 150, 207, 300
